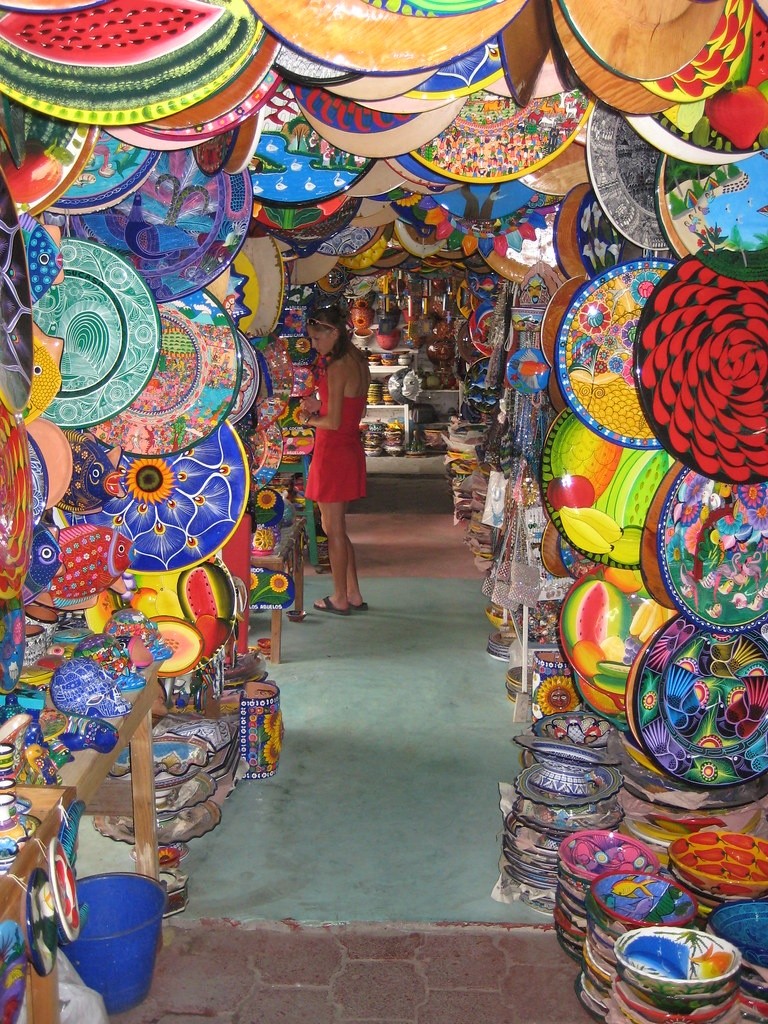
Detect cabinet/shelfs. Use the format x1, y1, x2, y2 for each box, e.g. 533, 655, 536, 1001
351, 326, 464, 455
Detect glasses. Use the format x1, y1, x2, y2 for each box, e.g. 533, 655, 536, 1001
306, 318, 336, 330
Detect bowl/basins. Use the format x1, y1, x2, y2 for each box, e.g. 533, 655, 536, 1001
1, 1, 768, 1023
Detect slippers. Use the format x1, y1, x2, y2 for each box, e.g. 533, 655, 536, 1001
349, 603, 368, 611
314, 597, 350, 616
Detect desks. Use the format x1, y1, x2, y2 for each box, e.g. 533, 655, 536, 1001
0, 655, 163, 1024
248, 517, 304, 664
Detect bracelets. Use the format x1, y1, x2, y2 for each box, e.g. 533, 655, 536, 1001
304, 415, 312, 422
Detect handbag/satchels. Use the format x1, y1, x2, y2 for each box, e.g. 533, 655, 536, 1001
483, 563, 542, 611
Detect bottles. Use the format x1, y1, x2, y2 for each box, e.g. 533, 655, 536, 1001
411, 430, 421, 452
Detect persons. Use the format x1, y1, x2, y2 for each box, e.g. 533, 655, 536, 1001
299, 305, 371, 614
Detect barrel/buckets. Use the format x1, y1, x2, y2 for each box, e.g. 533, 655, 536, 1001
52, 872, 169, 1016
